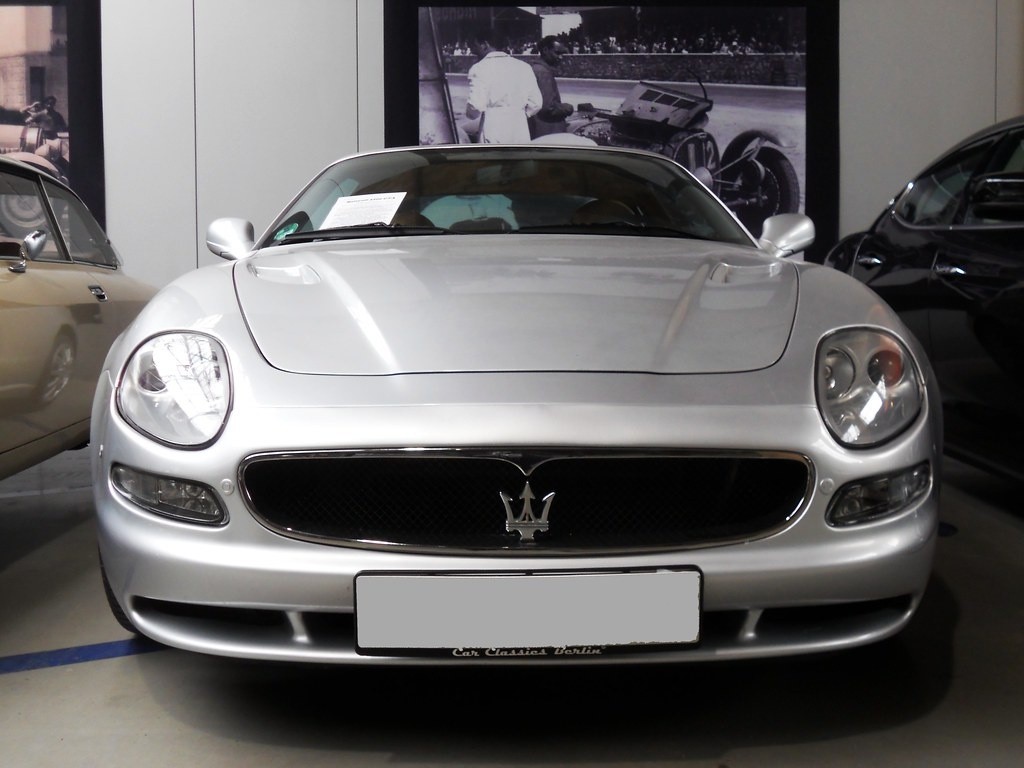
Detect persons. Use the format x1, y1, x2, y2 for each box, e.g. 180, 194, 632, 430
465, 27, 544, 145
527, 35, 574, 139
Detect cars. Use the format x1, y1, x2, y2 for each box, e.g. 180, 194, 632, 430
571, 78, 801, 240
825, 116, 1024, 494
1, 156, 162, 478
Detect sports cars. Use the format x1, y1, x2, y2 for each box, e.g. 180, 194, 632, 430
91, 141, 943, 669
